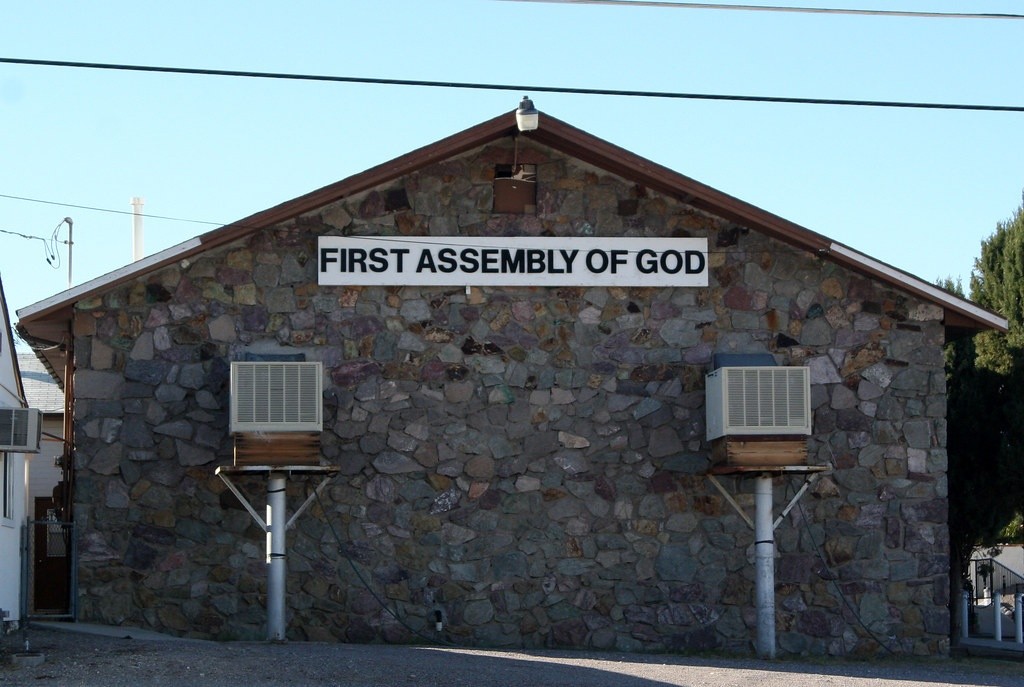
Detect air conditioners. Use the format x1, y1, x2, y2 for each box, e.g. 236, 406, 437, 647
0, 407, 43, 453
228, 361, 322, 435
705, 367, 810, 442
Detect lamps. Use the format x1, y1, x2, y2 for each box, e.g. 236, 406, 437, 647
512, 96, 539, 172
